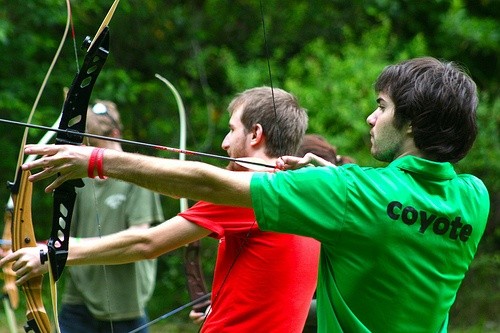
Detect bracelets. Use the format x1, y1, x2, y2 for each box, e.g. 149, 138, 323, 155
87, 147, 109, 180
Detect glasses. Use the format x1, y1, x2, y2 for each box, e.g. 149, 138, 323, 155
92, 102, 122, 136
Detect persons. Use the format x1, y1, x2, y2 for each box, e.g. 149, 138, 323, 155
56, 99, 166, 333
189, 134, 355, 333
0, 86, 325, 333
22, 58, 490, 333
